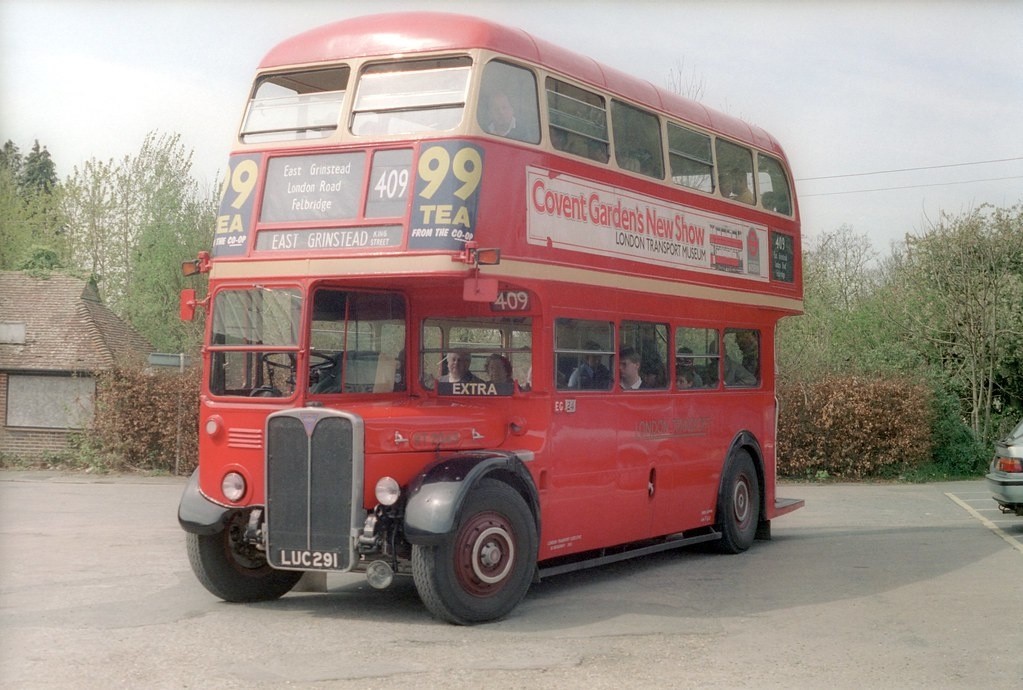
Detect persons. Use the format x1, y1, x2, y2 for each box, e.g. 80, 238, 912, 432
400, 331, 759, 391
483, 92, 754, 205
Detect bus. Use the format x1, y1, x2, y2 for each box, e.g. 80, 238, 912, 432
178, 9, 805, 629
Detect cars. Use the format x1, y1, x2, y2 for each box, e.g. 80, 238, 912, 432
986, 415, 1023, 517
